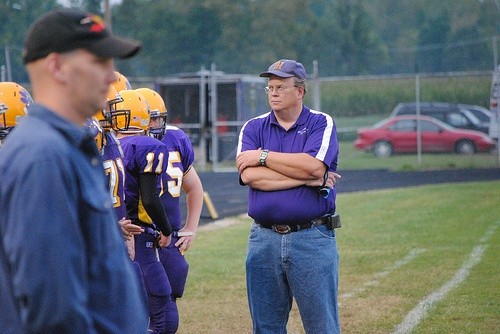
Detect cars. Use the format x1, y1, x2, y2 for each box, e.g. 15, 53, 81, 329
353, 114, 496, 160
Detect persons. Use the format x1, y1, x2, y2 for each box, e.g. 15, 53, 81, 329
135, 88, 203, 334
172, 112, 183, 124
0, 82, 33, 146
85, 71, 172, 334
216, 113, 229, 133
236, 59, 342, 334
0, 9, 146, 334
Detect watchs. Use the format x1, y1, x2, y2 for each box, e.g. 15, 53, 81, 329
259, 149, 269, 166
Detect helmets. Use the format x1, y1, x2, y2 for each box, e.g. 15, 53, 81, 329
134, 87, 168, 140
92, 70, 150, 132
0, 82, 34, 130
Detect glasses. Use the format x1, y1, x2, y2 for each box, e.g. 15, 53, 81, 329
263, 86, 297, 94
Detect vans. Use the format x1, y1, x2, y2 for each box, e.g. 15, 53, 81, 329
387, 102, 494, 137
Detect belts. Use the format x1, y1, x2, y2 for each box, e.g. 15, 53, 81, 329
254, 217, 327, 235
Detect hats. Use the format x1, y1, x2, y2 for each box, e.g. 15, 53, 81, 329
22, 8, 142, 66
259, 59, 306, 80
